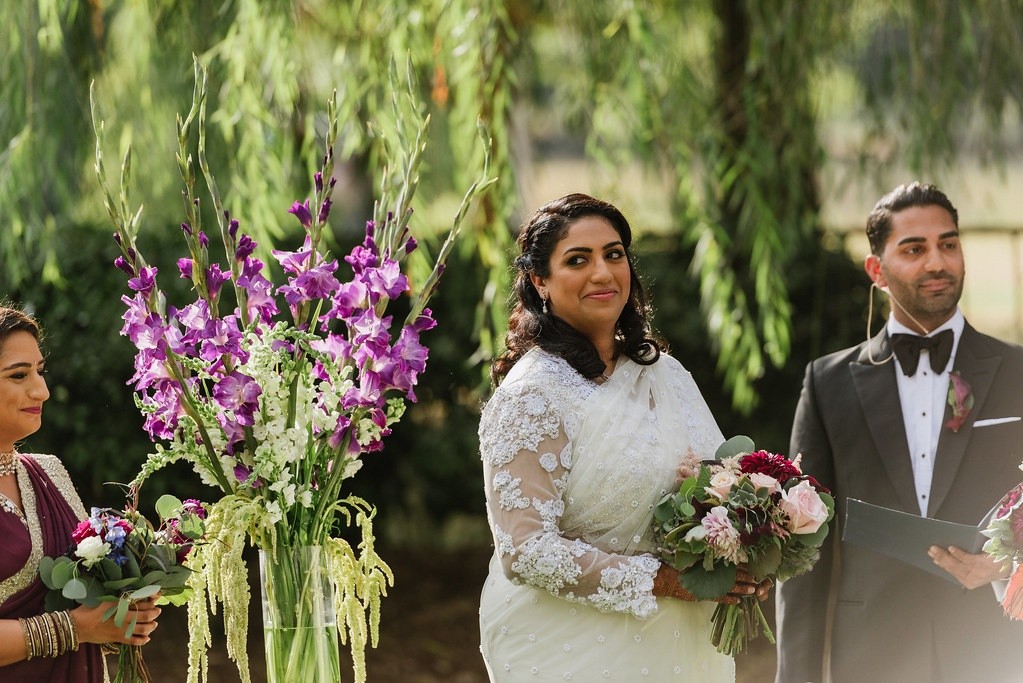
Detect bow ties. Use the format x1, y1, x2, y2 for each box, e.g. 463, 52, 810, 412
892, 329, 955, 377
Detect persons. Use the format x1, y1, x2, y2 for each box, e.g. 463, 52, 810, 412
775, 181, 1023, 683
477, 193, 777, 683
0, 306, 163, 683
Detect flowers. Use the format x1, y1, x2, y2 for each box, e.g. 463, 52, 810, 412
91, 49, 500, 683
40, 495, 206, 683
980, 483, 1023, 571
653, 435, 833, 656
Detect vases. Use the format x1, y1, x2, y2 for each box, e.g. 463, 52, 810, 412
255, 545, 345, 682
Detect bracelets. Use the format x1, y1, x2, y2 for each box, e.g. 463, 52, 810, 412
19, 610, 79, 661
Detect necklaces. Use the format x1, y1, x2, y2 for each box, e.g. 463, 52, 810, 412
0, 447, 16, 477
